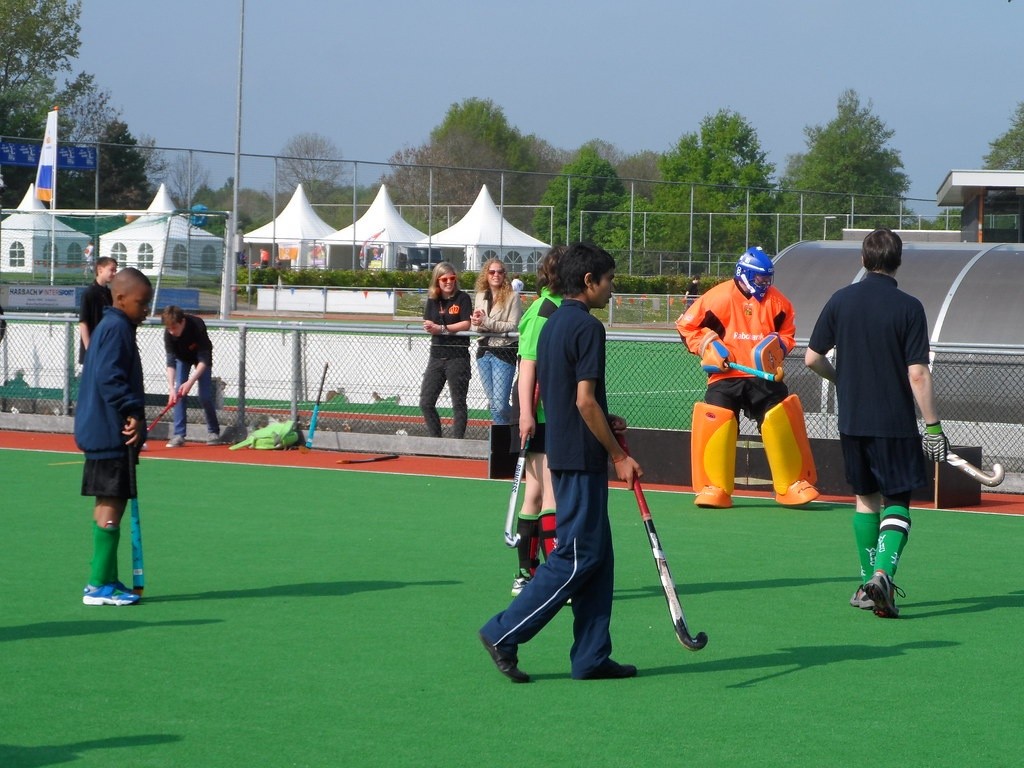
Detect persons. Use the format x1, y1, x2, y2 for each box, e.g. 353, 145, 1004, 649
72, 267, 155, 605
162, 306, 222, 449
682, 275, 700, 311
511, 245, 573, 606
84, 242, 94, 273
259, 247, 269, 270
421, 258, 525, 440
805, 229, 950, 617
677, 246, 820, 509
79, 257, 118, 365
479, 243, 645, 683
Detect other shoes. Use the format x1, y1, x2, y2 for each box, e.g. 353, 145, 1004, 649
572, 656, 637, 679
205, 433, 220, 445
775, 479, 820, 505
675, 320, 679, 324
510, 574, 529, 596
166, 435, 185, 447
694, 485, 734, 508
479, 634, 529, 682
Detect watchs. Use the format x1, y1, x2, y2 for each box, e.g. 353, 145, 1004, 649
443, 325, 449, 334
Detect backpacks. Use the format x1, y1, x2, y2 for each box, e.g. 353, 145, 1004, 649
228, 415, 298, 450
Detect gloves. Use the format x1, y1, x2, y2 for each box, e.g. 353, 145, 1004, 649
921, 430, 950, 463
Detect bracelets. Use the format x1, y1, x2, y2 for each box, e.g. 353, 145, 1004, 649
441, 325, 444, 333
613, 455, 628, 463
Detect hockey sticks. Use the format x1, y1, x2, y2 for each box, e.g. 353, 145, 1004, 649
503, 380, 540, 549
336, 453, 399, 464
140, 391, 182, 451
727, 360, 785, 383
921, 435, 1006, 487
299, 361, 329, 454
125, 419, 145, 601
612, 416, 710, 652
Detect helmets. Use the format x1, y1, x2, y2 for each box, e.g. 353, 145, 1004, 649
735, 247, 775, 301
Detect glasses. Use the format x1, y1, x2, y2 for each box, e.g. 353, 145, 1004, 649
488, 270, 505, 274
438, 275, 457, 282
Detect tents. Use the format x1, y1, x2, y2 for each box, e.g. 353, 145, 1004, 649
0, 183, 554, 281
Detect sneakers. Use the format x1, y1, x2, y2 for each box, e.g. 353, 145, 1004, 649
864, 570, 906, 618
850, 584, 874, 609
82, 581, 141, 606
